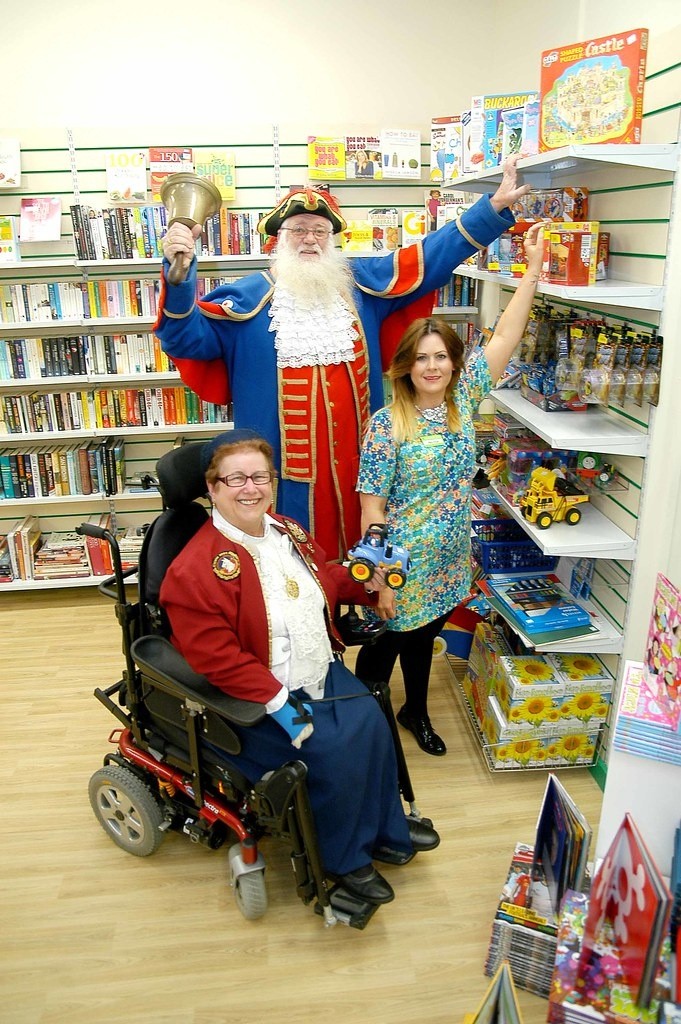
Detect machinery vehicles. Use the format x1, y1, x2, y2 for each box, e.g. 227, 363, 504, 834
347, 521, 413, 589
521, 466, 589, 530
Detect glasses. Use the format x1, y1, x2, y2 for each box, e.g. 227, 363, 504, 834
278, 224, 334, 240
215, 471, 273, 487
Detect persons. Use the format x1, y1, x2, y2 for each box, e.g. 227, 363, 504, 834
425, 190, 446, 231
159, 429, 440, 905
152, 154, 531, 663
354, 220, 547, 755
354, 149, 374, 179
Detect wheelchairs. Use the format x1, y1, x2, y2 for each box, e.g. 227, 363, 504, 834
76, 441, 435, 931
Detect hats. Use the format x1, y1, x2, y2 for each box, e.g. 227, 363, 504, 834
257, 188, 347, 236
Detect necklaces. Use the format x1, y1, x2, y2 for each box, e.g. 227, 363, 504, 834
413, 399, 448, 423
267, 541, 300, 600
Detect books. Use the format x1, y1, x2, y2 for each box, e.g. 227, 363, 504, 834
0, 72, 542, 586
465, 772, 681, 1024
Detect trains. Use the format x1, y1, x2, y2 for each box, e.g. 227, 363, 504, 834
576, 452, 616, 484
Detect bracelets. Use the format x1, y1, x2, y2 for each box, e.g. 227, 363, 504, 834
523, 270, 539, 284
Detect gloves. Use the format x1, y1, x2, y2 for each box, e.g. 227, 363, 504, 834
273, 693, 315, 750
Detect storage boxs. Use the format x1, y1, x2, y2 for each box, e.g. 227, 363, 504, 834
462, 621, 616, 768
342, 28, 650, 286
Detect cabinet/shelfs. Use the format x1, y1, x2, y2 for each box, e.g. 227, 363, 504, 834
0, 142, 479, 594
441, 141, 681, 774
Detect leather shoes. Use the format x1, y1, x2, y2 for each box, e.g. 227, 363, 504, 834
397, 705, 446, 757
326, 866, 396, 904
408, 818, 441, 851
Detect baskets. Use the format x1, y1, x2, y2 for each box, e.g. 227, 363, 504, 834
471, 518, 560, 574
